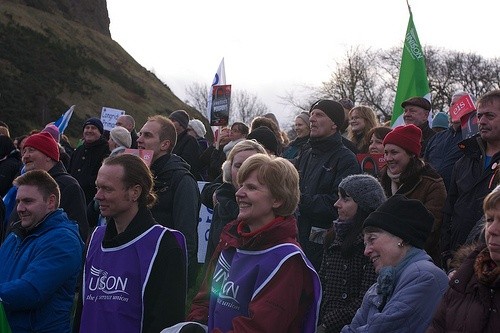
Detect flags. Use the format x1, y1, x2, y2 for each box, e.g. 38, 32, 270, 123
2, 105, 74, 220
390, 13, 432, 128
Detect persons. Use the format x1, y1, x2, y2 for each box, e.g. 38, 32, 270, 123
318, 174, 387, 333
188, 155, 322, 333
427, 185, 500, 333
0, 170, 85, 333
340, 89, 500, 264
341, 193, 449, 332
293, 98, 360, 272
0, 115, 138, 243
137, 109, 289, 299
71, 152, 187, 333
281, 111, 310, 164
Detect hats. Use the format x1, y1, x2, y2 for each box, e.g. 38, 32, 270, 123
41, 125, 59, 141
339, 175, 385, 212
384, 125, 423, 154
168, 110, 189, 128
247, 126, 278, 153
296, 111, 310, 127
312, 99, 344, 127
109, 126, 131, 147
83, 118, 103, 134
363, 193, 432, 246
23, 132, 60, 162
187, 119, 206, 137
401, 96, 430, 110
